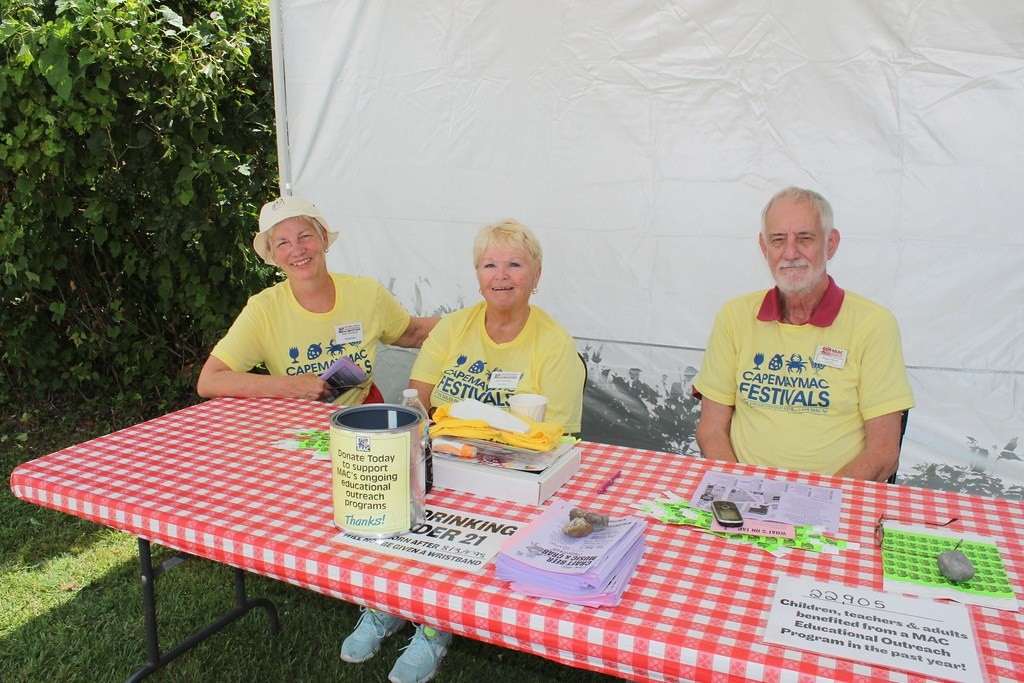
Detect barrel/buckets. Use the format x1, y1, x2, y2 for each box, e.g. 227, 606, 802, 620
329, 403, 427, 537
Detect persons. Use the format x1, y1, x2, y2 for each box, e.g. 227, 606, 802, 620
690, 186, 917, 484
339, 217, 586, 683
193, 196, 440, 407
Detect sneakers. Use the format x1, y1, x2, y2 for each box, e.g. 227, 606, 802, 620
341, 606, 406, 662
388, 620, 452, 683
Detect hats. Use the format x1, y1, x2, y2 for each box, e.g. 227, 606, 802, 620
253, 196, 339, 267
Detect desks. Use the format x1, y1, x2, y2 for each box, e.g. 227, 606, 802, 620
11, 397, 1024, 683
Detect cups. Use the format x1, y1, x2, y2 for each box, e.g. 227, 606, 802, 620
509, 394, 547, 423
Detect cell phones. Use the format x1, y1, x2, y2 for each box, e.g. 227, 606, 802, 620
711, 500, 743, 527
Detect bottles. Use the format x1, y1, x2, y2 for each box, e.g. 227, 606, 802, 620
401, 389, 429, 421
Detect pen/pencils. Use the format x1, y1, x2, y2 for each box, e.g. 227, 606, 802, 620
598, 470, 622, 494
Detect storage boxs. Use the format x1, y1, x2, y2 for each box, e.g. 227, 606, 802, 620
432, 446, 582, 508
